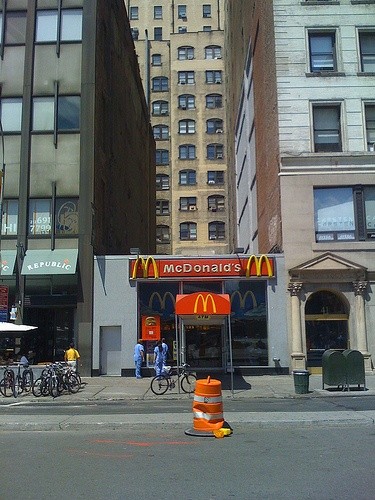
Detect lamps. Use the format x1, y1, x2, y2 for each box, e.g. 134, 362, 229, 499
130, 248, 141, 255
233, 248, 244, 254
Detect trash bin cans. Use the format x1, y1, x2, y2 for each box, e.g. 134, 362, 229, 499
291, 371, 311, 393
321, 349, 367, 391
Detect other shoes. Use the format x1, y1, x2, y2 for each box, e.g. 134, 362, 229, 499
137, 376, 142, 379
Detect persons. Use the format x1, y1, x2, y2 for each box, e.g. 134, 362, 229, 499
153, 338, 168, 381
17, 352, 28, 363
64, 343, 80, 382
133, 339, 145, 379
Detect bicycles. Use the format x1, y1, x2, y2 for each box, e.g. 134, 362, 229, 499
31, 362, 81, 398
11, 362, 33, 398
0, 365, 15, 398
150, 363, 198, 395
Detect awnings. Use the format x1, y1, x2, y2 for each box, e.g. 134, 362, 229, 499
0, 249, 78, 275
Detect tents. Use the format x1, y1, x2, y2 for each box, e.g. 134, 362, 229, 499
0, 322, 39, 334
